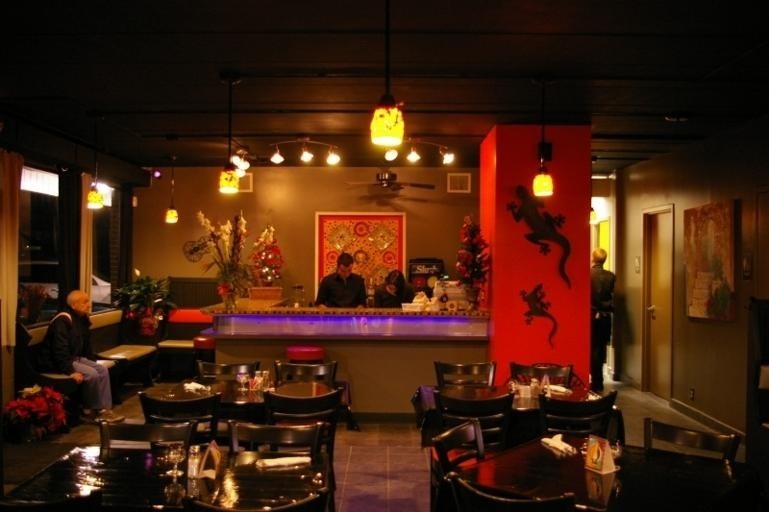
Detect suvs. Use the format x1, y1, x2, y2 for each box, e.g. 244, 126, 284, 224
16, 259, 113, 308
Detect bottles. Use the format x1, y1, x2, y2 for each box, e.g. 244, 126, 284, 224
530, 377, 539, 399
253, 369, 270, 391
187, 445, 201, 477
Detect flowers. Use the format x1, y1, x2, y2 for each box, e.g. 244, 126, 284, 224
453, 212, 491, 289
249, 223, 284, 279
197, 210, 281, 292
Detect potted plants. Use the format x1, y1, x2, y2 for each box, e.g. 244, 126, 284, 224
112, 270, 180, 344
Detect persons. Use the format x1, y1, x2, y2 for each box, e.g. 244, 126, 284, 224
41, 289, 126, 424
695, 218, 726, 278
314, 253, 368, 309
374, 270, 417, 309
589, 247, 617, 394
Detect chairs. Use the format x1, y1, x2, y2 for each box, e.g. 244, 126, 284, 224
2, 357, 352, 512
409, 360, 744, 511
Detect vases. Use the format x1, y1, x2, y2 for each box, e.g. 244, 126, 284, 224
464, 286, 481, 303
220, 292, 242, 312
251, 270, 274, 287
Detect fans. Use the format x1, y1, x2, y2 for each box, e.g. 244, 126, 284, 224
345, 161, 436, 192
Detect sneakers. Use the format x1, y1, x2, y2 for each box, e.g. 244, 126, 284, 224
94, 410, 125, 423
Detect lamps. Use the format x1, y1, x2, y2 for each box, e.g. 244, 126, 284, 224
214, 70, 243, 198
366, 0, 408, 151
84, 115, 105, 212
530, 64, 556, 200
376, 136, 459, 167
265, 139, 349, 168
230, 138, 254, 177
163, 153, 180, 226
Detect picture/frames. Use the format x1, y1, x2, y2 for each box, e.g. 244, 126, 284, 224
313, 210, 408, 303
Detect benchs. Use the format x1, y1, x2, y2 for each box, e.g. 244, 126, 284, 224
157, 337, 195, 358
93, 342, 158, 403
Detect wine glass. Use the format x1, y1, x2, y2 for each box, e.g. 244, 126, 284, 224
164, 475, 185, 504
166, 445, 184, 476
237, 365, 249, 392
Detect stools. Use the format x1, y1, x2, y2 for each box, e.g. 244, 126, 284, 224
193, 334, 216, 351
286, 344, 325, 363
